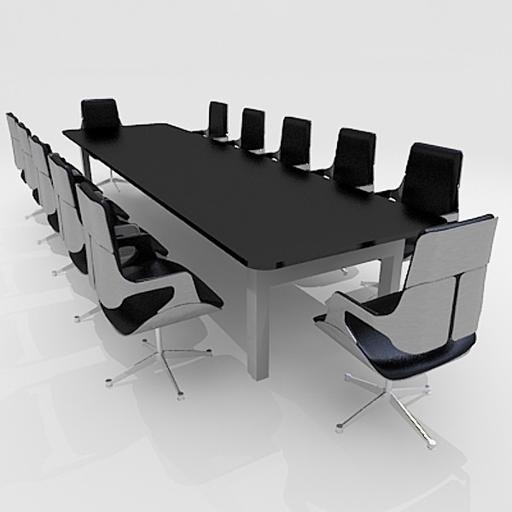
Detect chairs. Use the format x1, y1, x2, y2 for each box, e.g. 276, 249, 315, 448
312, 213, 499, 448
7, 97, 464, 404
